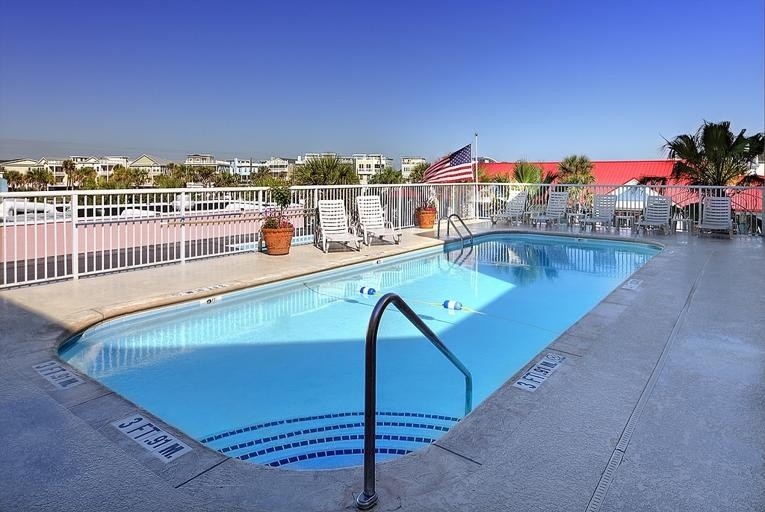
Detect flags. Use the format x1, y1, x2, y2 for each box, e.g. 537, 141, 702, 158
421, 144, 474, 185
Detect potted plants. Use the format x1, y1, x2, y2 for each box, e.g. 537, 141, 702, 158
315, 195, 402, 254
414, 187, 437, 229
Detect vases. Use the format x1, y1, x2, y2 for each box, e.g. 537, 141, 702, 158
261, 227, 294, 256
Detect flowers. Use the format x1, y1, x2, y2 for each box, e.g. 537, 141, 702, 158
262, 184, 292, 228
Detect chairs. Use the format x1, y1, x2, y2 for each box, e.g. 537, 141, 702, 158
489, 191, 569, 226
696, 196, 731, 239
581, 195, 617, 231
634, 196, 672, 235
